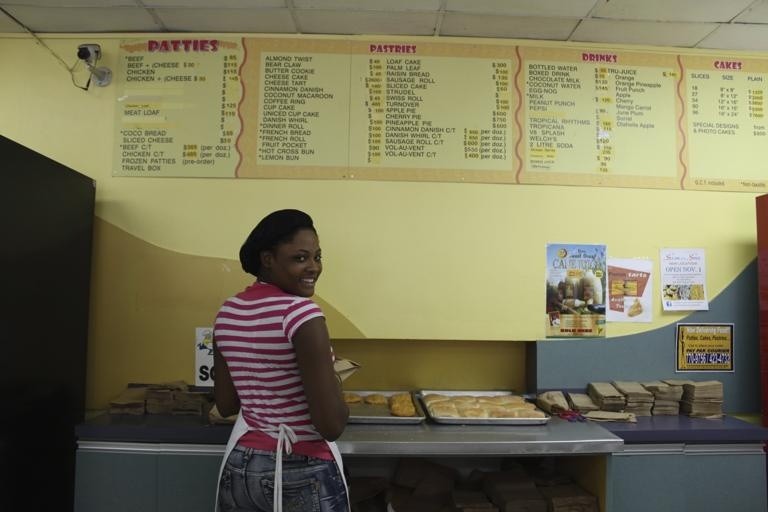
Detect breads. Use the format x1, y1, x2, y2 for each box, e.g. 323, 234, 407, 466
423, 391, 547, 421
341, 390, 416, 418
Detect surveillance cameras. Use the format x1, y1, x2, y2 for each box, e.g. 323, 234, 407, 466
77, 44, 100, 61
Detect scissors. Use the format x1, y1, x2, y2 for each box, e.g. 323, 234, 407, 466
560, 410, 586, 423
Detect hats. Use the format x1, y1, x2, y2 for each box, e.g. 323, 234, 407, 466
239, 210, 312, 274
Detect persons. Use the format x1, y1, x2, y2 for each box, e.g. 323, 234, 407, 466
212, 210, 351, 512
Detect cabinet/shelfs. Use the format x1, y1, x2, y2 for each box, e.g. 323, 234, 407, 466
610, 442, 768, 512
